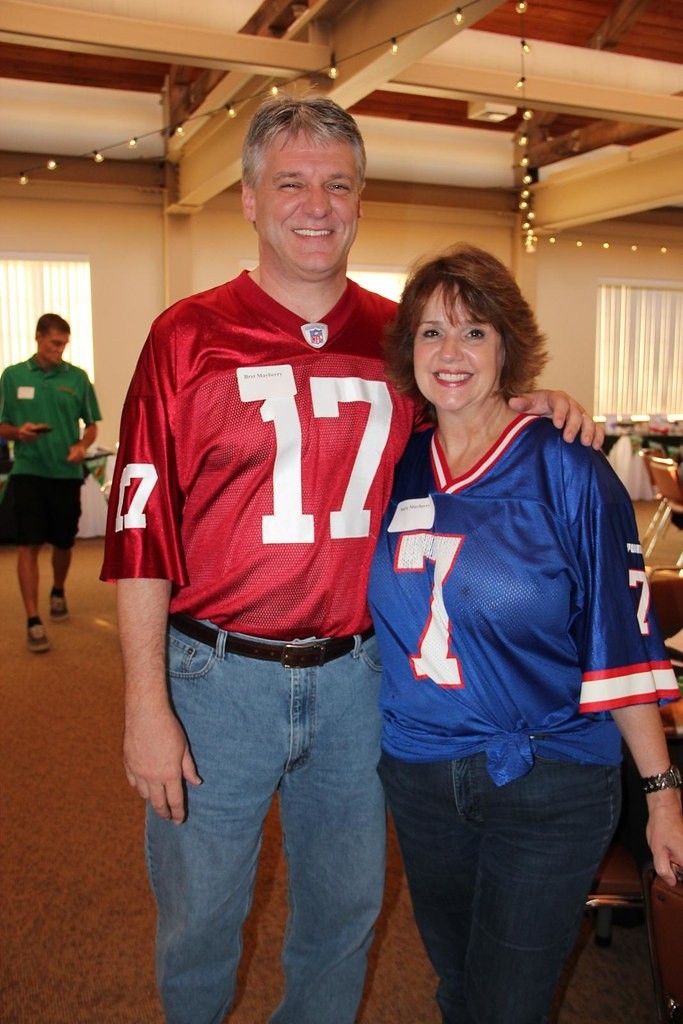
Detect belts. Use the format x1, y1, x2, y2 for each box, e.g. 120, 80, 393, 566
168, 612, 376, 668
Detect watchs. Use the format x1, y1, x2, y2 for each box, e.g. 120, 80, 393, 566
642, 765, 682, 794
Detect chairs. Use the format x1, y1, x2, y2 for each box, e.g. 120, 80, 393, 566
641, 456, 683, 558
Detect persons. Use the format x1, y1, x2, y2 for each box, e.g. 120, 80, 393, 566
0, 313, 102, 653
98, 97, 605, 1024
366, 244, 683, 1024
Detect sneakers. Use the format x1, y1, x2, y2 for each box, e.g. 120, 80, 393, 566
28, 624, 49, 651
49, 595, 69, 621
